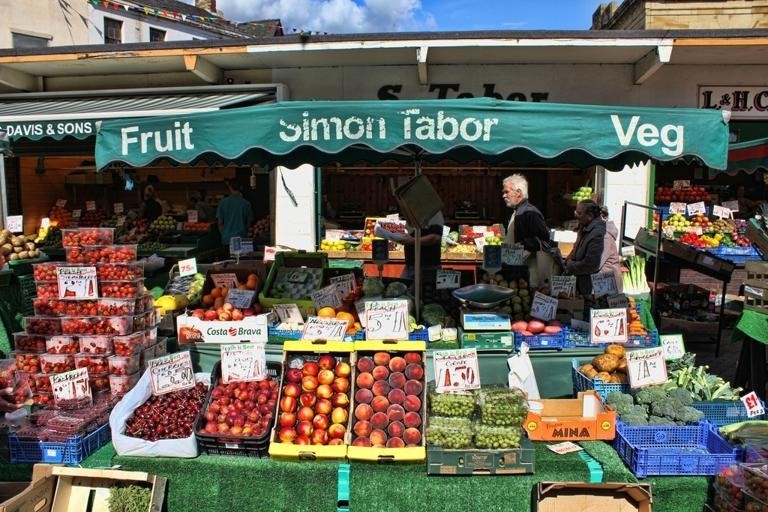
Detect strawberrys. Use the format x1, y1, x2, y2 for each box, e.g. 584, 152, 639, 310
705, 464, 768, 512
0, 228, 156, 402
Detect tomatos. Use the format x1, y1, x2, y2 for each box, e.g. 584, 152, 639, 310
362, 241, 372, 252
511, 319, 563, 338
678, 231, 708, 249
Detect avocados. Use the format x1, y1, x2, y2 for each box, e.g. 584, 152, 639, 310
344, 241, 362, 253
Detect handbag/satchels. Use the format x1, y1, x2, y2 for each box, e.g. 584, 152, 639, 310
526, 249, 562, 289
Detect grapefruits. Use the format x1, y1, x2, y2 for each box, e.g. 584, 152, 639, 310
156, 290, 189, 316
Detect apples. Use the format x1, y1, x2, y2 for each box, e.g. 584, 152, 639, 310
572, 185, 593, 201
150, 215, 176, 230
485, 235, 503, 246
667, 212, 692, 234
193, 302, 255, 321
364, 219, 377, 239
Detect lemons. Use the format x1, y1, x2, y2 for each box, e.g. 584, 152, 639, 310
320, 239, 346, 252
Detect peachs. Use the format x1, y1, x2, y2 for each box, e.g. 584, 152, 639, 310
352, 352, 423, 449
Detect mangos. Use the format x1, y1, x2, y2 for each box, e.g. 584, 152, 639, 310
690, 212, 714, 233
714, 219, 736, 234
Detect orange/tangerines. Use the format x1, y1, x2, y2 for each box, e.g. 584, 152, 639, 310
315, 305, 364, 335
185, 221, 211, 230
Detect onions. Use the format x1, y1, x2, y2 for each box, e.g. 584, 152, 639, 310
654, 186, 713, 203
731, 230, 752, 248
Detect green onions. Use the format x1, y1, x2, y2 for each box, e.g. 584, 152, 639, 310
621, 254, 651, 296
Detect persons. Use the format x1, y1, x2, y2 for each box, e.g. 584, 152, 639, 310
214, 178, 254, 256
590, 203, 618, 272
393, 208, 444, 293
496, 172, 549, 277
139, 184, 171, 226
562, 198, 603, 295
729, 184, 762, 219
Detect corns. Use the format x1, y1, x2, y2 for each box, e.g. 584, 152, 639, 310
718, 420, 768, 444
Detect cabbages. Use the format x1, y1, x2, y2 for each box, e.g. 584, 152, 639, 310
361, 275, 457, 331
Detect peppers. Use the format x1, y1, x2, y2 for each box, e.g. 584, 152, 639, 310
701, 232, 740, 249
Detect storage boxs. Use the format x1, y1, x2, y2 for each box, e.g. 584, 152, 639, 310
551, 228, 577, 259
0, 463, 65, 512
657, 313, 719, 343
523, 387, 617, 446
636, 215, 768, 289
159, 251, 328, 343
319, 218, 505, 260
269, 341, 425, 464
425, 436, 539, 474
531, 481, 653, 512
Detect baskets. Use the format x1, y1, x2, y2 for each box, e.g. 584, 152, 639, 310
192, 358, 282, 458
514, 325, 659, 349
7, 422, 112, 466
571, 358, 765, 479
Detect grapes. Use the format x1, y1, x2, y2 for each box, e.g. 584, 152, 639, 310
379, 220, 405, 252
733, 218, 749, 233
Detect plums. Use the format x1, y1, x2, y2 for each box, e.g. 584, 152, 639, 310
280, 355, 350, 446
199, 373, 279, 444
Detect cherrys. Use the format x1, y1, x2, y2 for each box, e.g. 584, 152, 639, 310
1, 388, 119, 444
127, 380, 207, 444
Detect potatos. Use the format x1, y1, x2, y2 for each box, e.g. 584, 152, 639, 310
577, 344, 630, 383
479, 272, 570, 320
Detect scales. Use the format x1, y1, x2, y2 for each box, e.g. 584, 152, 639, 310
338, 200, 366, 218
455, 200, 481, 220
451, 283, 519, 330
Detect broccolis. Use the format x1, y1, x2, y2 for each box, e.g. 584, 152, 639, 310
603, 385, 706, 427
